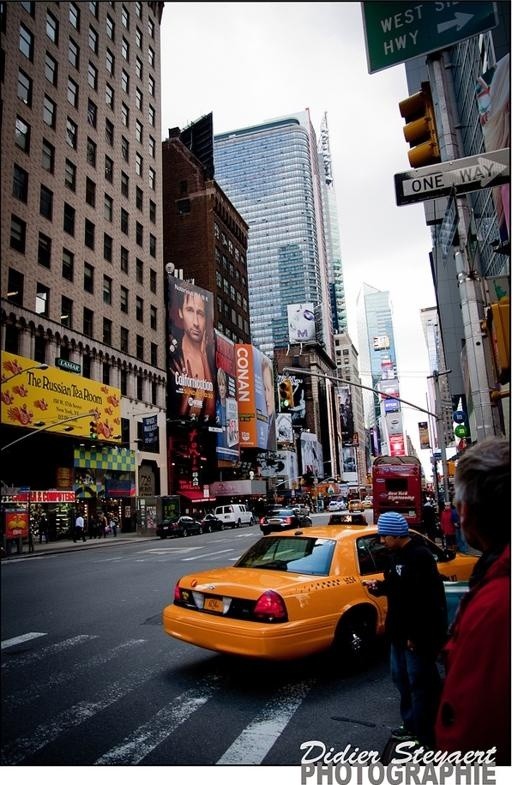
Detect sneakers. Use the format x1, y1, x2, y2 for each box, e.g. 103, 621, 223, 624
392, 726, 416, 740
406, 741, 431, 756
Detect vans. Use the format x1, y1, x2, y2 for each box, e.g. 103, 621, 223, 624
213, 504, 254, 528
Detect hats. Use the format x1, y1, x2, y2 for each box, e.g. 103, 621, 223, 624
378, 512, 408, 536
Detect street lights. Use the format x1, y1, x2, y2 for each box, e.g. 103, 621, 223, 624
314, 460, 332, 492
0, 363, 48, 385
271, 475, 303, 496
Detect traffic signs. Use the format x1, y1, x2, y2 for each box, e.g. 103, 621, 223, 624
395, 147, 510, 208
359, 0, 500, 73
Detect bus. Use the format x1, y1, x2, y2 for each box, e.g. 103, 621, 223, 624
372, 455, 423, 529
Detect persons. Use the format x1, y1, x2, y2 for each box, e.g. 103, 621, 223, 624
215, 367, 241, 461
171, 287, 213, 416
308, 448, 322, 474
422, 486, 469, 554
261, 461, 285, 477
434, 438, 510, 765
260, 359, 275, 451
363, 511, 448, 749
36, 510, 118, 543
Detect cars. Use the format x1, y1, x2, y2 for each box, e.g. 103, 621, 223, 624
192, 513, 225, 533
259, 509, 313, 534
328, 514, 368, 526
154, 514, 202, 538
161, 524, 480, 661
256, 480, 371, 514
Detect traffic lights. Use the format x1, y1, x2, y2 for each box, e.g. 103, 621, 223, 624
278, 379, 295, 409
88, 421, 98, 441
485, 296, 511, 386
398, 81, 439, 168
